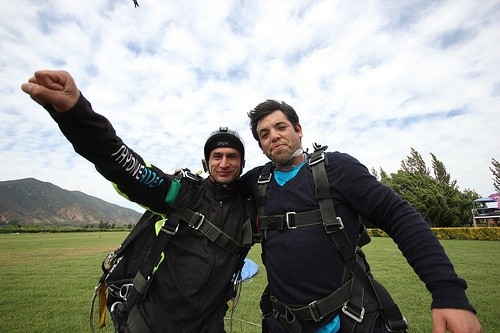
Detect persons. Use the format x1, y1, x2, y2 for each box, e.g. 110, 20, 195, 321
232, 100, 484, 333
21, 69, 259, 333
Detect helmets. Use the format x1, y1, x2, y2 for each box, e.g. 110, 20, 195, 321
200, 126, 248, 170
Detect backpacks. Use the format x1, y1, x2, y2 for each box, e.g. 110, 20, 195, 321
94, 166, 200, 326
